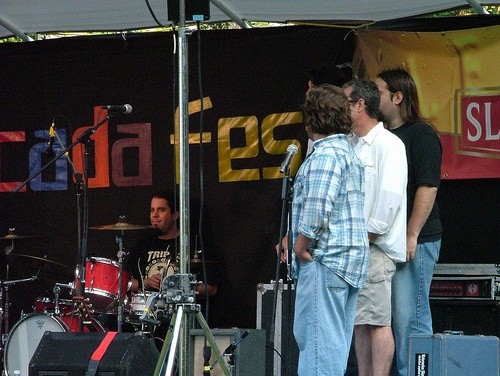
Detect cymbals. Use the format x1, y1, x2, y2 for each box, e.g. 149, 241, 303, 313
0, 229, 39, 239
186, 255, 218, 264
7, 250, 71, 270
89, 220, 156, 231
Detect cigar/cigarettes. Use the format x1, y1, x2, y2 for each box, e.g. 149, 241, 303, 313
280, 260, 283, 263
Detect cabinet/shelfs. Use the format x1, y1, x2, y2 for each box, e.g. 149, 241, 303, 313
256, 282, 299, 375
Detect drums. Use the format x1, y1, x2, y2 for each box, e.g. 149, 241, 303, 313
33, 295, 95, 315
3, 308, 106, 375
70, 256, 132, 316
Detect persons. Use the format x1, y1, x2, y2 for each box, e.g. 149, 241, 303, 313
372, 65, 442, 376
105, 191, 219, 354
274, 82, 370, 376
339, 79, 408, 376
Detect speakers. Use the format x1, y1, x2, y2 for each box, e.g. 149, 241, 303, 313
167, 0, 210, 22
28, 331, 167, 376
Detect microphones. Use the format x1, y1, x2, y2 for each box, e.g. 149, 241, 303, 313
102, 104, 133, 114
202, 346, 211, 376
279, 143, 298, 173
45, 118, 56, 154
